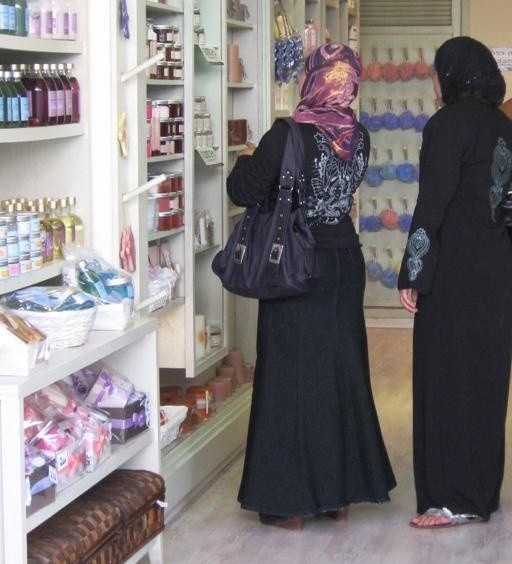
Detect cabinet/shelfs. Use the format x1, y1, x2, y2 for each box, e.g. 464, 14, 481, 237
266, 2, 359, 130
2, 0, 265, 563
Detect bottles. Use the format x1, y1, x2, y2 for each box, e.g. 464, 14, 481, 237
306, 17, 319, 55
146, 171, 184, 232
147, 95, 214, 158
149, 23, 183, 79
0, 62, 81, 129
208, 321, 224, 349
0, 196, 84, 280
0, 0, 79, 41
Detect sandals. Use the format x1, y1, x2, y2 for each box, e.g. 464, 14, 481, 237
407, 503, 479, 528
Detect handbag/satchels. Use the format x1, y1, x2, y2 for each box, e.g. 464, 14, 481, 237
211, 116, 316, 300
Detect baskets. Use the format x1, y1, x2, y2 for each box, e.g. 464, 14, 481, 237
27, 469, 166, 563
4, 254, 134, 347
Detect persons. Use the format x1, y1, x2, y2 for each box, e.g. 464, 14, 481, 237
398, 37, 512, 526
225, 42, 398, 530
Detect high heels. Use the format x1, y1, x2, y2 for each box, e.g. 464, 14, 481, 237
259, 501, 351, 532
